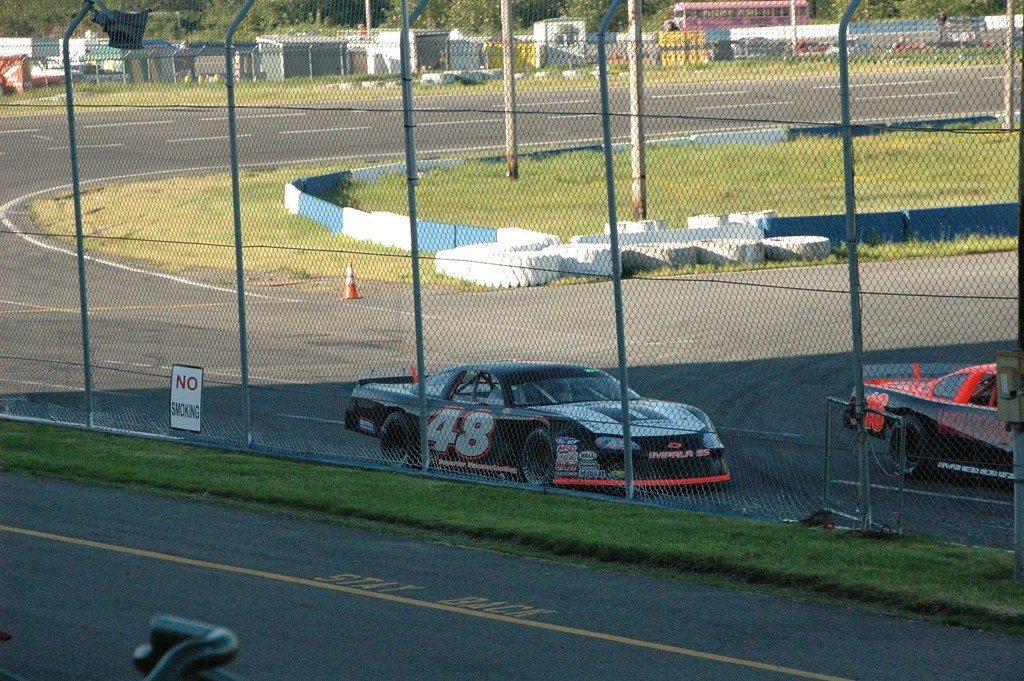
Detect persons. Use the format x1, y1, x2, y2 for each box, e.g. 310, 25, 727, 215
183, 72, 222, 84
412, 64, 431, 74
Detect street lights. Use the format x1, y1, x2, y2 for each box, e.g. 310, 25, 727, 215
53, 0, 154, 428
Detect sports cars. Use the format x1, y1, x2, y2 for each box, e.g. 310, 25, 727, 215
844, 361, 1017, 490
345, 360, 733, 498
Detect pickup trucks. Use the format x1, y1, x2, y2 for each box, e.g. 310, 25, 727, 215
65, 58, 132, 83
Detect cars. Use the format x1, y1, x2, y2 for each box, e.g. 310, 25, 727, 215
722, 30, 1024, 66
22, 56, 80, 90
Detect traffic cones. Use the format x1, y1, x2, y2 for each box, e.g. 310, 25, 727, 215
338, 258, 364, 299
